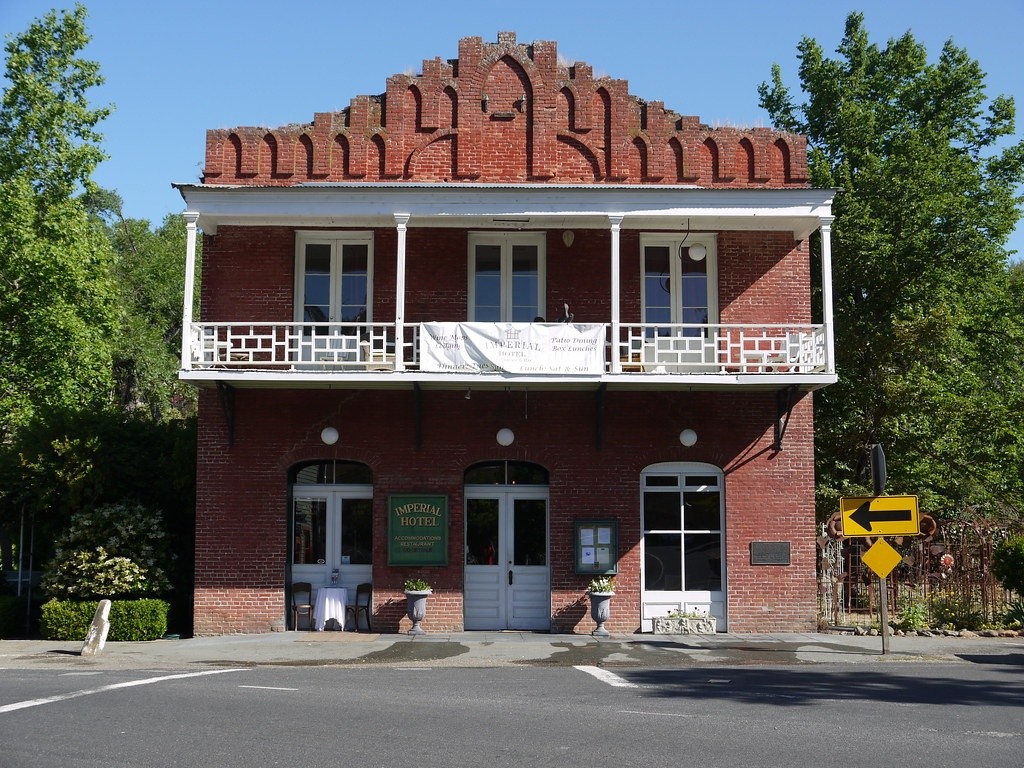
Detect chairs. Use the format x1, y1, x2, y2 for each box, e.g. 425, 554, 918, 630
291, 582, 314, 632
344, 583, 372, 632
359, 333, 807, 372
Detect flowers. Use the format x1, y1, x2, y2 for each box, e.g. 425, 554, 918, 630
587, 575, 616, 594
666, 607, 712, 619
403, 577, 432, 591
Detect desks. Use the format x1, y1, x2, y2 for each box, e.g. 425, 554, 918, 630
734, 353, 772, 373
202, 341, 234, 369
605, 343, 629, 347
312, 585, 348, 631
220, 354, 260, 369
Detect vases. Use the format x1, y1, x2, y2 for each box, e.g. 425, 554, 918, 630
652, 616, 717, 635
588, 594, 614, 636
404, 592, 430, 635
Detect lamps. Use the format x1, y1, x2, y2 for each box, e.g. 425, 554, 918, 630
679, 429, 698, 447
320, 427, 340, 445
496, 428, 514, 446
678, 218, 707, 264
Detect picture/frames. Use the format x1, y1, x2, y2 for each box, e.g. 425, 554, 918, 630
574, 519, 619, 574
